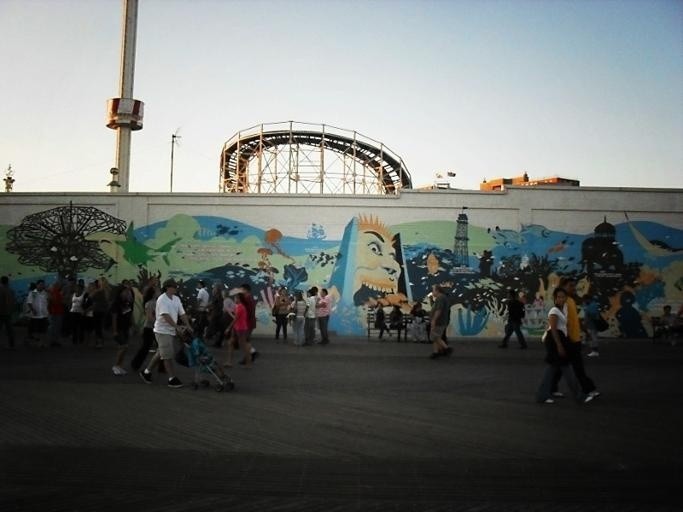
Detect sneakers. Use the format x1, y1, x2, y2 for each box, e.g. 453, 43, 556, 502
379, 335, 455, 359
29, 338, 258, 387
500, 343, 601, 404
277, 337, 331, 346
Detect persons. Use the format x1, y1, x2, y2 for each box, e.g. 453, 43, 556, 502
654, 305, 683, 346
497, 290, 527, 350
372, 283, 455, 359
0, 270, 258, 390
549, 279, 601, 397
272, 286, 330, 347
537, 287, 593, 405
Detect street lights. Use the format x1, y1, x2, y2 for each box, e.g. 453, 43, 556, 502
3, 164, 15, 193
446, 171, 458, 189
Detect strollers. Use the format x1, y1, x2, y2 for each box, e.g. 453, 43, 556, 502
175, 325, 234, 392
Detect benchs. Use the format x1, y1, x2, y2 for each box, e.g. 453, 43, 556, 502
650, 314, 672, 343
366, 312, 433, 343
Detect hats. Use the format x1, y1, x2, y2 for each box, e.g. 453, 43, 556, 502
163, 278, 181, 288
